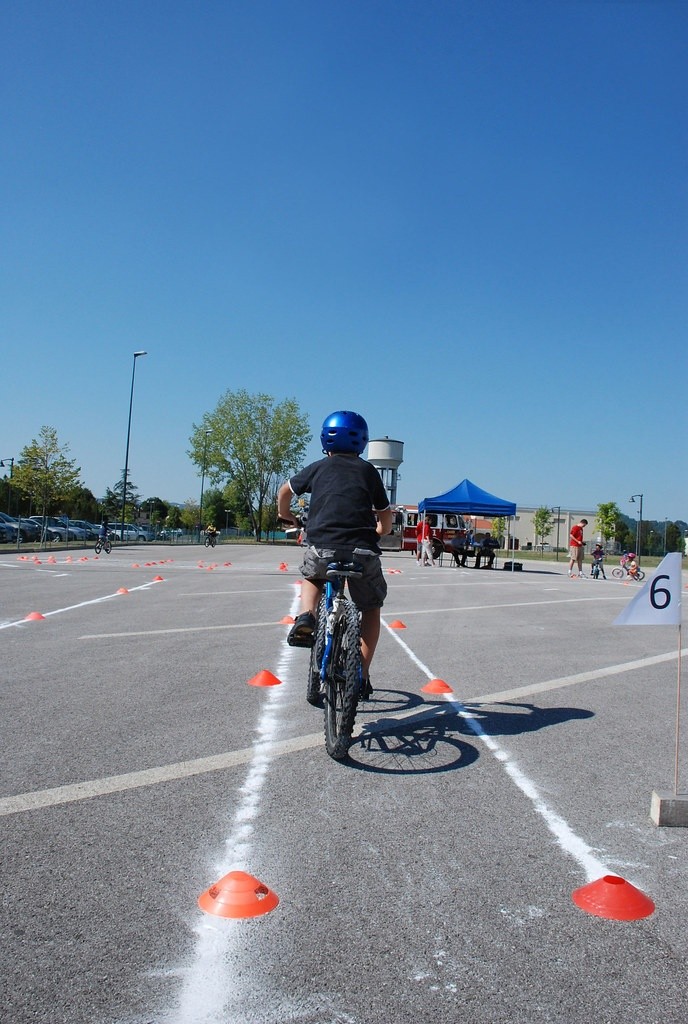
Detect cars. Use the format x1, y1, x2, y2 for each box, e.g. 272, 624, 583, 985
94, 525, 121, 543
0, 512, 77, 543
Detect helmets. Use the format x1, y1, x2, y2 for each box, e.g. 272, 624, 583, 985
596, 543, 602, 549
627, 553, 635, 558
320, 411, 369, 454
102, 521, 108, 526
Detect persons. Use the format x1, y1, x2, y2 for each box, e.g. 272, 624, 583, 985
98, 521, 109, 549
627, 553, 640, 582
277, 411, 392, 702
568, 519, 588, 578
205, 523, 217, 541
451, 529, 501, 569
415, 517, 441, 568
591, 543, 607, 579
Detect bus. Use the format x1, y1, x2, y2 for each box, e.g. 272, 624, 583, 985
396, 505, 468, 559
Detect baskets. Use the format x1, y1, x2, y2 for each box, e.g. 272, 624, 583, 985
620, 560, 626, 565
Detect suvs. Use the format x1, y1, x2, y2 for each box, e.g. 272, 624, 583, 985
104, 523, 154, 542
69, 520, 109, 541
28, 516, 86, 542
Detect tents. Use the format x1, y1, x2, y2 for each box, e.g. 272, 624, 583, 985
418, 479, 516, 572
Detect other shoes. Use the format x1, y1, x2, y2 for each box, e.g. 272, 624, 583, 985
457, 563, 461, 567
603, 576, 606, 579
630, 578, 633, 580
577, 574, 588, 579
567, 573, 573, 578
488, 562, 491, 568
432, 565, 438, 568
637, 579, 640, 582
425, 563, 431, 566
474, 566, 480, 569
591, 573, 594, 575
416, 561, 421, 566
461, 564, 467, 567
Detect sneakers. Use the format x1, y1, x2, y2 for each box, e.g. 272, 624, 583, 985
360, 679, 373, 694
287, 610, 317, 644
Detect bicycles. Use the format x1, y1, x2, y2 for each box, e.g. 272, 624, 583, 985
203, 530, 218, 547
275, 510, 402, 759
95, 532, 113, 554
589, 553, 608, 579
612, 557, 645, 580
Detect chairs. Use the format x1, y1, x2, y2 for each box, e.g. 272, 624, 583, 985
449, 543, 467, 568
477, 546, 494, 567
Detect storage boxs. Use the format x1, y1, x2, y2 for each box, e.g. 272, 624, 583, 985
505, 562, 523, 571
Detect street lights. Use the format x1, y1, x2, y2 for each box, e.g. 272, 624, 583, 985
553, 506, 565, 562
0, 458, 21, 517
118, 351, 155, 542
628, 494, 643, 566
224, 509, 231, 535
197, 430, 215, 543
